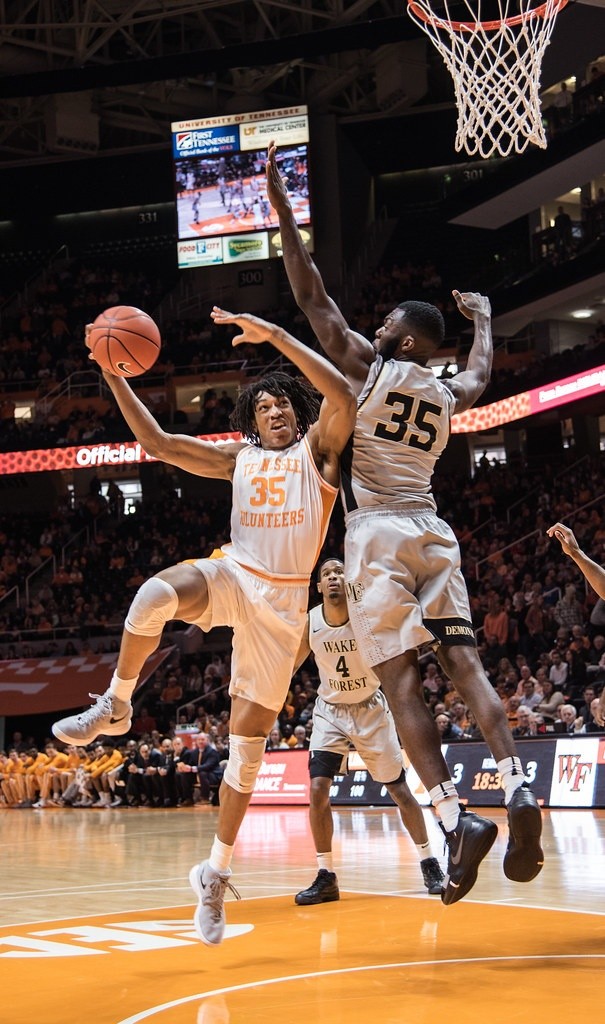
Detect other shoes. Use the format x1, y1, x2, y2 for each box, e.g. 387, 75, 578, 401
1, 796, 218, 809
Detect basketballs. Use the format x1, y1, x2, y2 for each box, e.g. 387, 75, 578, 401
89, 305, 162, 378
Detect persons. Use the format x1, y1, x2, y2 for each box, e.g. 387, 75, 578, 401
590, 68, 605, 108
176, 154, 308, 230
555, 83, 572, 126
0, 188, 605, 808
267, 141, 543, 904
546, 522, 605, 601
52, 307, 356, 945
292, 558, 446, 904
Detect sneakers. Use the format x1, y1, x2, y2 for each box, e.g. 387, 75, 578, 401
189, 860, 241, 947
420, 858, 446, 894
295, 869, 340, 905
52, 693, 134, 746
438, 803, 498, 905
503, 781, 545, 884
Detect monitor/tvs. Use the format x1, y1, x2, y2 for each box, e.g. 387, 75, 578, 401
537, 722, 567, 734
